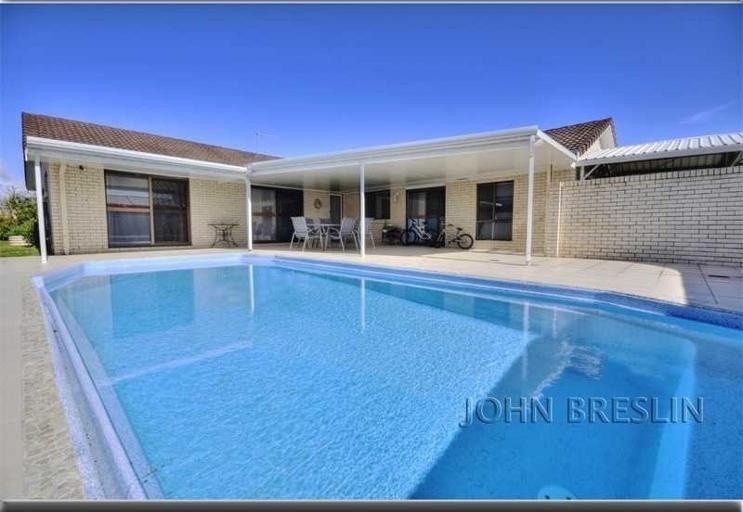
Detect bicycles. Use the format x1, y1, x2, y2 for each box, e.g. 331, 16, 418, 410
434, 215, 474, 250
399, 218, 439, 246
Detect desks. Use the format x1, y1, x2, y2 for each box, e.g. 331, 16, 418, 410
207, 223, 241, 247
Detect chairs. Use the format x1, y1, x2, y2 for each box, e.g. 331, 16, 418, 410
289, 216, 376, 253
